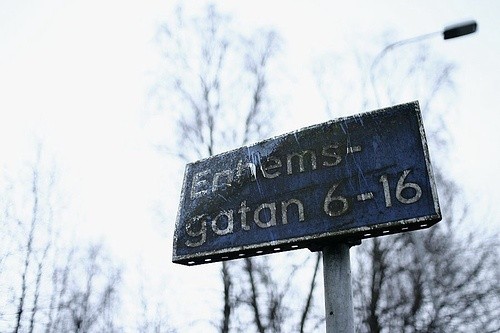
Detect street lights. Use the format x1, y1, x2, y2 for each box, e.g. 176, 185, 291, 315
368, 18, 477, 333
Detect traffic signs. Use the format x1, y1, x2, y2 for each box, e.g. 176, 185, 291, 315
172, 101, 441, 267
